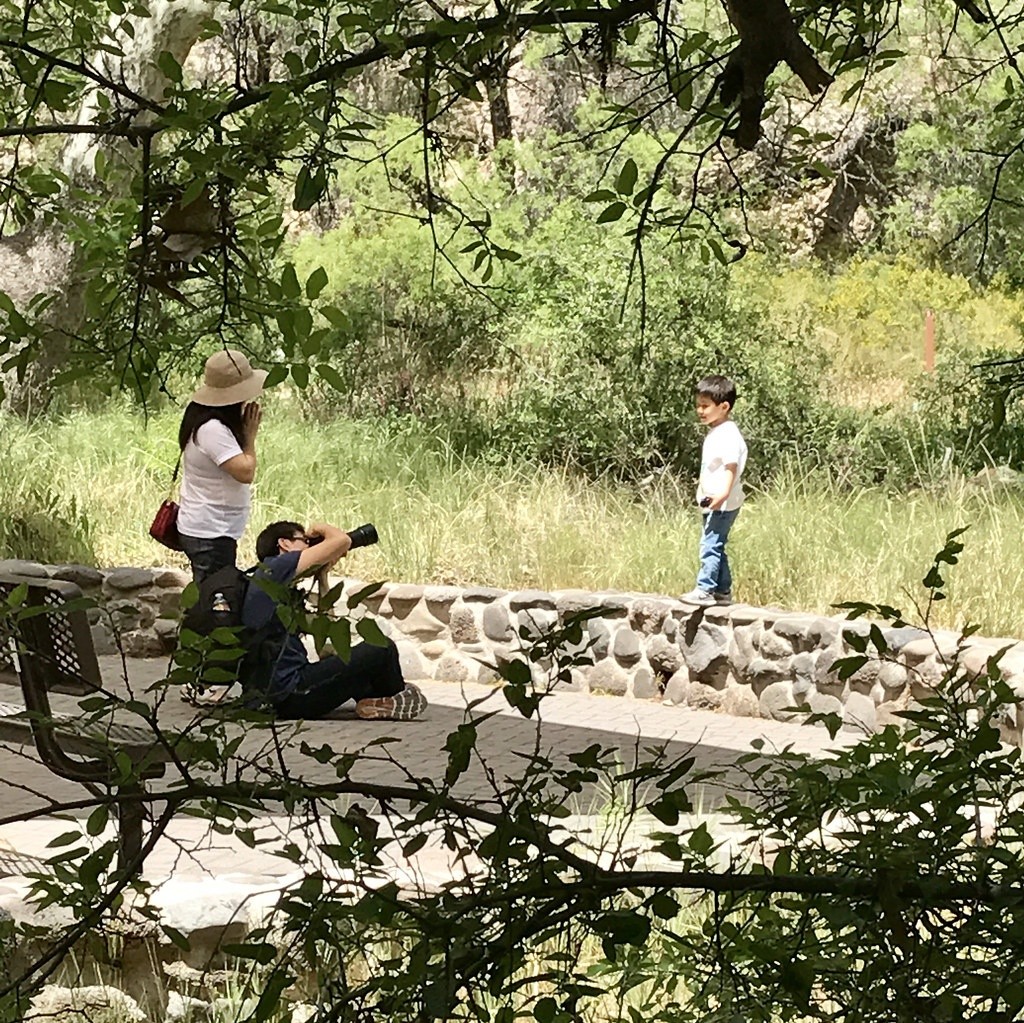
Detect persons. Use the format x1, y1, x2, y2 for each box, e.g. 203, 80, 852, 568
242, 520, 427, 721
679, 374, 748, 606
175, 350, 269, 707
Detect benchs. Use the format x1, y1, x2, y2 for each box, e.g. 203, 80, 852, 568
0, 573, 219, 873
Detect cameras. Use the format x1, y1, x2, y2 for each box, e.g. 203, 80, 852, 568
310, 523, 379, 552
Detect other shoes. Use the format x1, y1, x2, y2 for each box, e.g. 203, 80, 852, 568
355, 688, 428, 721
404, 682, 422, 692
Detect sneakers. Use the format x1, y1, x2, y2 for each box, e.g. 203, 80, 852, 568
678, 587, 717, 607
713, 590, 732, 606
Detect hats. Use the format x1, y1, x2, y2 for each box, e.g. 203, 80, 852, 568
191, 350, 269, 406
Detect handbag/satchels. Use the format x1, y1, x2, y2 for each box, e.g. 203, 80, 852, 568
149, 499, 184, 552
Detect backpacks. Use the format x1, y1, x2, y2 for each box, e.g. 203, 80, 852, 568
178, 563, 298, 686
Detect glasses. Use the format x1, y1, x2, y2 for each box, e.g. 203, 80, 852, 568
276, 533, 311, 550
